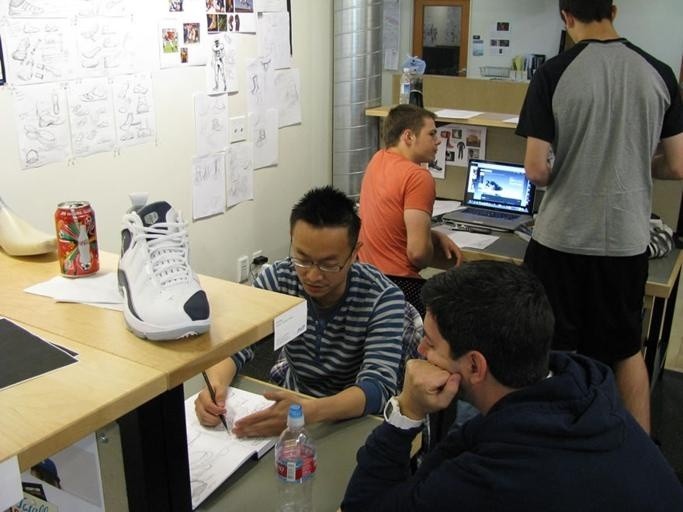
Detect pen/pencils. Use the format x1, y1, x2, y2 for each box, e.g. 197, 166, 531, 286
202, 370, 229, 432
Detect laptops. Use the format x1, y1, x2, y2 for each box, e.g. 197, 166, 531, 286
442, 159, 536, 231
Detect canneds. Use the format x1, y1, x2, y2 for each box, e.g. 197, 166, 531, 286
55, 200, 99, 278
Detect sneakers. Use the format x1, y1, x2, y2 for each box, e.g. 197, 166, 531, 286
117, 191, 211, 341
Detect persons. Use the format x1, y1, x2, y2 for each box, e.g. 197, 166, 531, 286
334, 258, 683, 509
209, 37, 228, 93
514, 0, 681, 440
29, 456, 61, 489
193, 188, 421, 438
356, 103, 466, 313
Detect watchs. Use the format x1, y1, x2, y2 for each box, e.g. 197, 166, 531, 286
381, 391, 428, 430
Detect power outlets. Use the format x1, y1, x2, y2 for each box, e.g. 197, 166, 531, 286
250, 251, 263, 273
238, 256, 249, 283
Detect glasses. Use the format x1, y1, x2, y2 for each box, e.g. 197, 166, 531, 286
285, 248, 354, 273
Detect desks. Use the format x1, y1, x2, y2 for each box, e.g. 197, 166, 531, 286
429, 218, 683, 398
184, 369, 423, 512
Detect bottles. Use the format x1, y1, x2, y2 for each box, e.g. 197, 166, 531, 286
399, 68, 412, 105
275, 403, 318, 512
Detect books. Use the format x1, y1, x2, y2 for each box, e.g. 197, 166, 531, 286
183, 382, 280, 510
